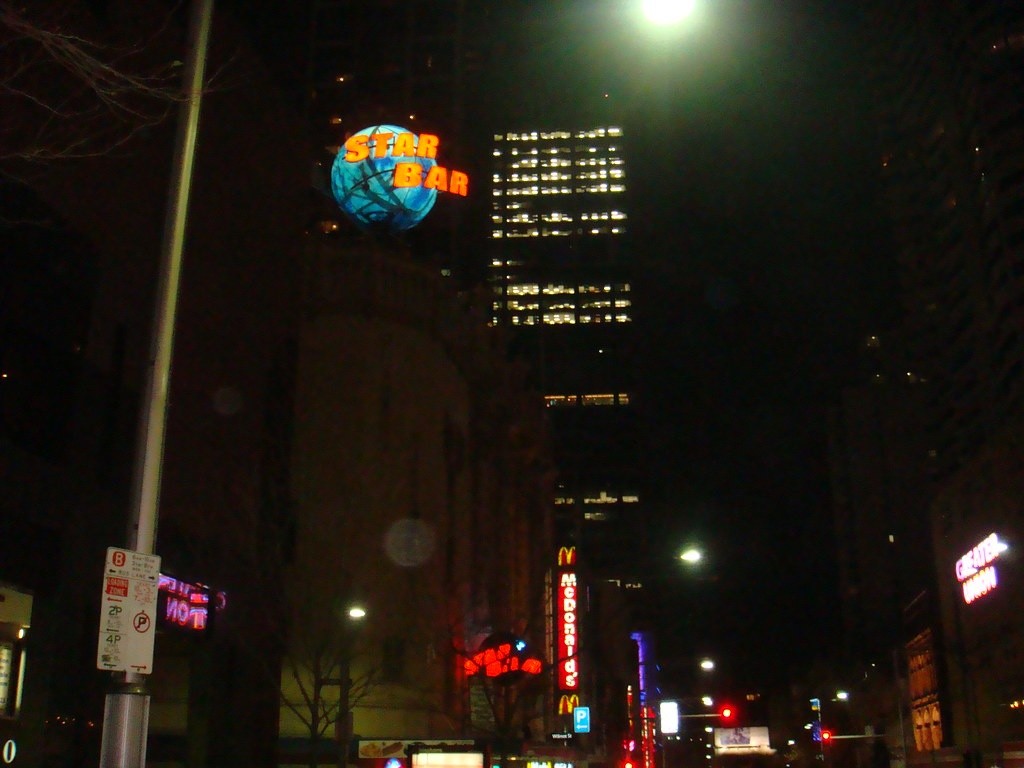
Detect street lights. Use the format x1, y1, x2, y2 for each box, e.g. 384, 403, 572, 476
809, 697, 824, 767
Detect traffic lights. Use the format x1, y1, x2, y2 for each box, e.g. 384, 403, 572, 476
822, 730, 830, 752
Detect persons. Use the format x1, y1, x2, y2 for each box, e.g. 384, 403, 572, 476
517, 717, 869, 768
869, 725, 901, 768
